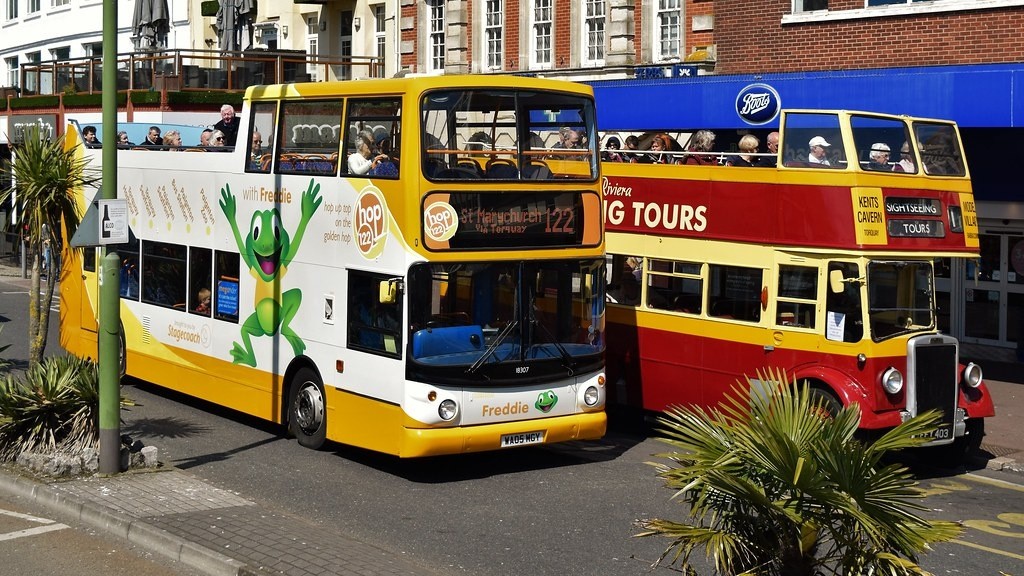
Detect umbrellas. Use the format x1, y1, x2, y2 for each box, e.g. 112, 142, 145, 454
132, 0, 169, 67
212, 0, 257, 70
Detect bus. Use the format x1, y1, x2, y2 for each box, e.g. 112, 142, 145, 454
58, 73, 607, 460
391, 108, 996, 474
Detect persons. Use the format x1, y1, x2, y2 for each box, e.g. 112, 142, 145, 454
42, 219, 62, 281
80, 104, 930, 359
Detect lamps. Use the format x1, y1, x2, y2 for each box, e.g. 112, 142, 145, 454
355, 18, 360, 31
320, 21, 326, 32
283, 25, 288, 35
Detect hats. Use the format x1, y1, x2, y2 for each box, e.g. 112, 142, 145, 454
808, 135, 831, 147
376, 134, 391, 146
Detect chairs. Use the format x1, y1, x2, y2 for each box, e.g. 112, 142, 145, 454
302, 155, 331, 171
256, 154, 285, 171
330, 151, 352, 173
370, 156, 400, 177
452, 158, 483, 178
485, 159, 519, 179
522, 160, 553, 182
262, 158, 295, 172
424, 157, 447, 178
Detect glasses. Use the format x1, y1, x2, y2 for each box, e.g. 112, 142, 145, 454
216, 137, 226, 141
253, 140, 262, 144
173, 138, 180, 141
607, 143, 618, 148
